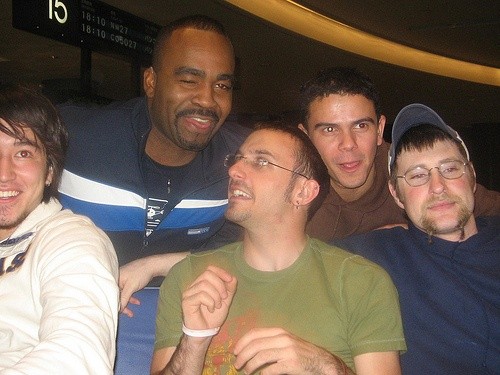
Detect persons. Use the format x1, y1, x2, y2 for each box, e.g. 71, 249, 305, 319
1, 91, 120, 375
296, 79, 500, 242
51, 22, 254, 317
150, 118, 407, 375
117, 104, 500, 374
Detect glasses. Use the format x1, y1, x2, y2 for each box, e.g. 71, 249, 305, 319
224, 154, 309, 180
395, 160, 468, 186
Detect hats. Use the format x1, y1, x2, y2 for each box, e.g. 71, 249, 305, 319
388, 103, 470, 175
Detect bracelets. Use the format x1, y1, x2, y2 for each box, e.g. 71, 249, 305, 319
181, 324, 222, 340
